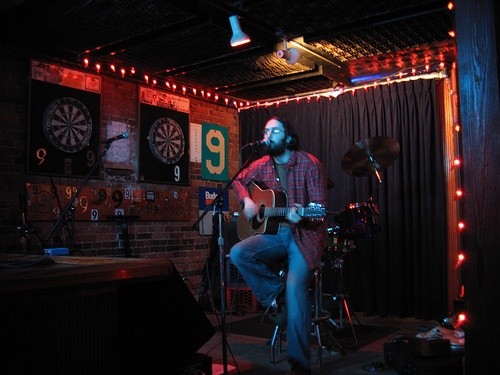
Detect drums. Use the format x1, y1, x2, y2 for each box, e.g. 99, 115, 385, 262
324, 225, 344, 256
344, 198, 383, 244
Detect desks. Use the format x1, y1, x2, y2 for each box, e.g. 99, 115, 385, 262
0, 253, 217, 375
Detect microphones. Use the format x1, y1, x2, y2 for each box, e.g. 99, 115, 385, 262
100, 133, 129, 143
245, 138, 271, 147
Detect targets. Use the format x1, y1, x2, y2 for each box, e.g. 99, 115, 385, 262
148, 117, 185, 165
41, 96, 93, 153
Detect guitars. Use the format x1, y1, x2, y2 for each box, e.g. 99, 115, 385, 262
236, 178, 329, 241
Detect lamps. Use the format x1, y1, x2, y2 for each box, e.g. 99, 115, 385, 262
277, 38, 299, 64
229, 16, 251, 47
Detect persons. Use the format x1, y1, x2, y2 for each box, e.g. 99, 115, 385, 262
229, 116, 328, 375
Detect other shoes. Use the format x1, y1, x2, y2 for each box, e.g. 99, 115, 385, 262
262, 292, 286, 313
287, 365, 311, 375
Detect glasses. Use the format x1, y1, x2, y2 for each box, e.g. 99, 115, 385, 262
264, 128, 287, 134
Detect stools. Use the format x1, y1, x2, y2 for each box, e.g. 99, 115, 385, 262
269, 255, 347, 368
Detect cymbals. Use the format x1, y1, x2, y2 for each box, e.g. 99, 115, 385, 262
339, 135, 402, 176
